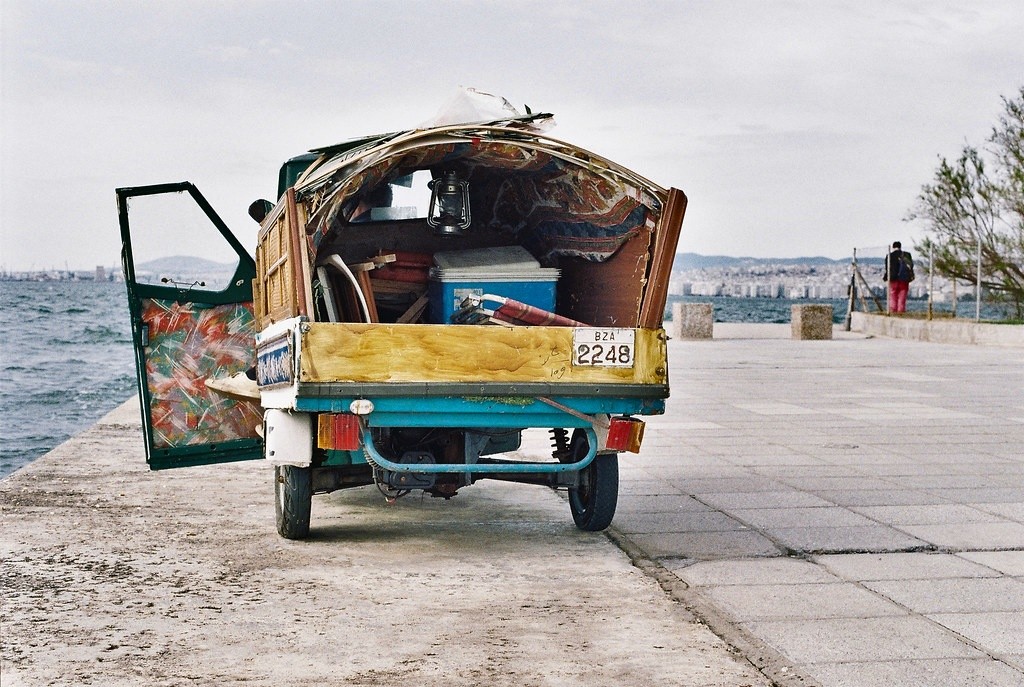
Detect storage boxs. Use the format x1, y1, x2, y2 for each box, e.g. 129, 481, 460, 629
426, 245, 563, 326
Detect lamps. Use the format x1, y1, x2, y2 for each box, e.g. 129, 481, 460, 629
427, 161, 472, 238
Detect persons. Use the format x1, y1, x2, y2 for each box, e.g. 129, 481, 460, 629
883, 241, 915, 313
351, 183, 392, 223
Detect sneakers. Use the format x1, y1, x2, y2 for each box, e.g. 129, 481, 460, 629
255, 424, 265, 437
203, 372, 260, 398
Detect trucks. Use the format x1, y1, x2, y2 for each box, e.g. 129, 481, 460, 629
115, 129, 690, 540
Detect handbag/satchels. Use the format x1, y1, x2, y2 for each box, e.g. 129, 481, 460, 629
898, 252, 911, 278
